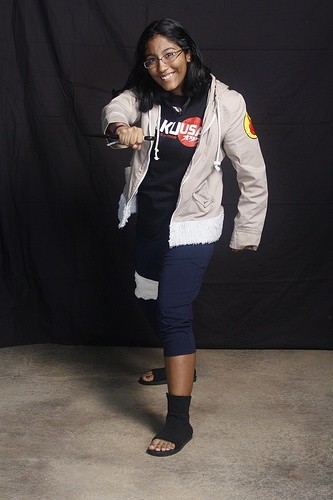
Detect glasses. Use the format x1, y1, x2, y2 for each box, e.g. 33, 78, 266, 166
142, 47, 183, 70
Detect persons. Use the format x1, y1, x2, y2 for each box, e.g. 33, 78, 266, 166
101, 17, 269, 457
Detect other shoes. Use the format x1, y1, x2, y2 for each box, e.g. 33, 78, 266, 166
147, 393, 193, 457
138, 361, 197, 385
